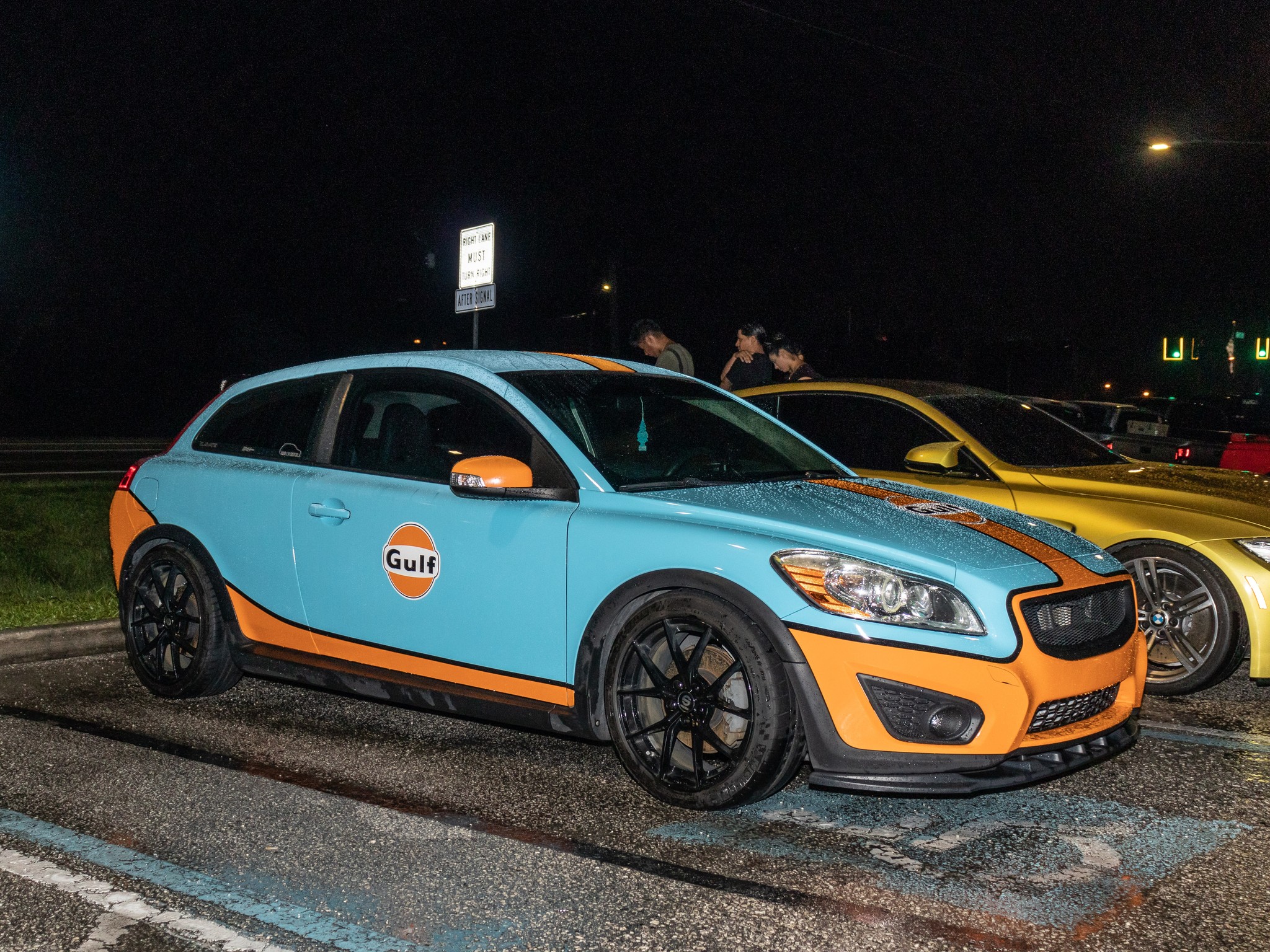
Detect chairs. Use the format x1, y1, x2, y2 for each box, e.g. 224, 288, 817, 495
378, 402, 462, 481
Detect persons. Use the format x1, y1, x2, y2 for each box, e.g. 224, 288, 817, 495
628, 318, 695, 377
768, 332, 818, 380
720, 323, 777, 393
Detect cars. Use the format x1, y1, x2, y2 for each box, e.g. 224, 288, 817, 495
99, 345, 1154, 814
1016, 393, 1270, 478
720, 381, 1270, 698
